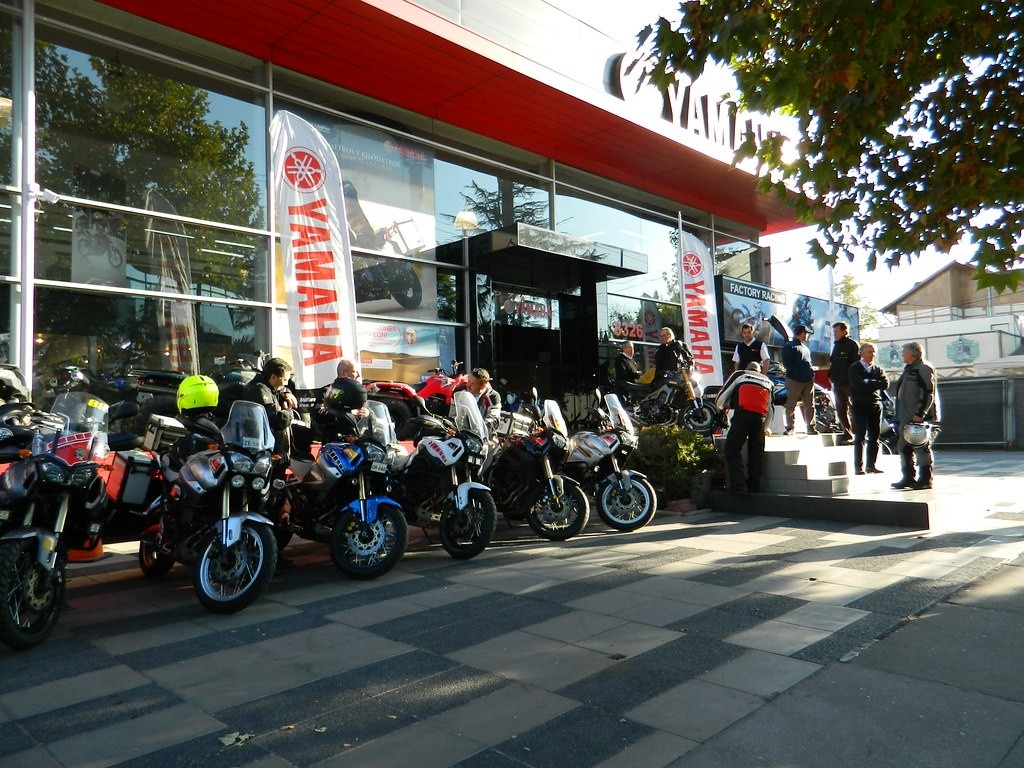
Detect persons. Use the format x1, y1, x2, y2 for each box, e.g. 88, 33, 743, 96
449, 368, 501, 483
732, 324, 770, 374
714, 361, 775, 493
891, 342, 941, 490
244, 357, 296, 575
782, 325, 819, 435
314, 360, 361, 408
848, 343, 884, 474
615, 341, 642, 385
653, 327, 693, 379
826, 321, 861, 445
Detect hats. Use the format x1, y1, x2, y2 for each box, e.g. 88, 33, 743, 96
795, 324, 814, 334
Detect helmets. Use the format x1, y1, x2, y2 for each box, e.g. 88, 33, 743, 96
324, 377, 368, 425
176, 374, 219, 416
903, 421, 931, 448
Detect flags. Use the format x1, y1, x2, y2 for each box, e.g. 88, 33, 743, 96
678, 229, 723, 386
268, 109, 363, 384
141, 188, 199, 377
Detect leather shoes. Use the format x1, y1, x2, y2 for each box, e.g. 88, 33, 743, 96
866, 464, 883, 473
856, 467, 865, 474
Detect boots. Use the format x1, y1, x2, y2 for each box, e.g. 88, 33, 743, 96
910, 467, 933, 489
891, 469, 917, 488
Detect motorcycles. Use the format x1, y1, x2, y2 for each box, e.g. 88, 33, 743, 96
0, 360, 658, 650
593, 340, 723, 437
770, 361, 898, 456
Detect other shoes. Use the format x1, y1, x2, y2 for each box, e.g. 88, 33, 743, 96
747, 485, 758, 492
726, 483, 745, 493
807, 425, 819, 435
784, 428, 795, 435
